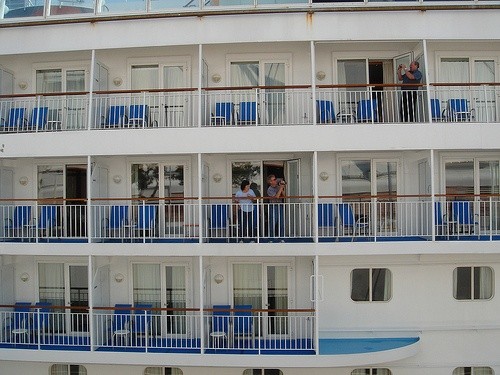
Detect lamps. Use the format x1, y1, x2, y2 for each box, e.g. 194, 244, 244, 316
214, 274, 224, 284
211, 74, 220, 83
114, 273, 124, 283
320, 172, 329, 180
213, 173, 223, 183
316, 71, 326, 80
113, 77, 122, 86
113, 175, 121, 183
20, 272, 30, 282
19, 176, 29, 186
19, 81, 28, 90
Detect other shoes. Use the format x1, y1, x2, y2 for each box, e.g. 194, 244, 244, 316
240, 241, 243, 243
250, 241, 255, 243
269, 241, 273, 243
281, 240, 285, 243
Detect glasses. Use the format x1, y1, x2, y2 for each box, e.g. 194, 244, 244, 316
271, 179, 276, 181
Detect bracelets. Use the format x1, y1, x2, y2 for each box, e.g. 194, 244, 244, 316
404, 68, 407, 70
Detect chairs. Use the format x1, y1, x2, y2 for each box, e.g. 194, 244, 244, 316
317, 99, 379, 123
3, 302, 54, 344
434, 201, 474, 235
212, 304, 254, 348
111, 304, 153, 347
212, 101, 258, 125
0, 107, 48, 131
431, 99, 476, 121
318, 204, 371, 241
100, 105, 150, 127
101, 206, 156, 238
3, 205, 56, 237
205, 205, 257, 236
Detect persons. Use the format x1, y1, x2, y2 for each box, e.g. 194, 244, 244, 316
397, 61, 422, 122
235, 181, 257, 243
267, 175, 286, 243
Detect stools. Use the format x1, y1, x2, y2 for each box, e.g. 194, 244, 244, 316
11, 329, 29, 343
112, 330, 131, 347
123, 224, 137, 242
228, 223, 240, 243
22, 224, 36, 241
210, 332, 228, 349
